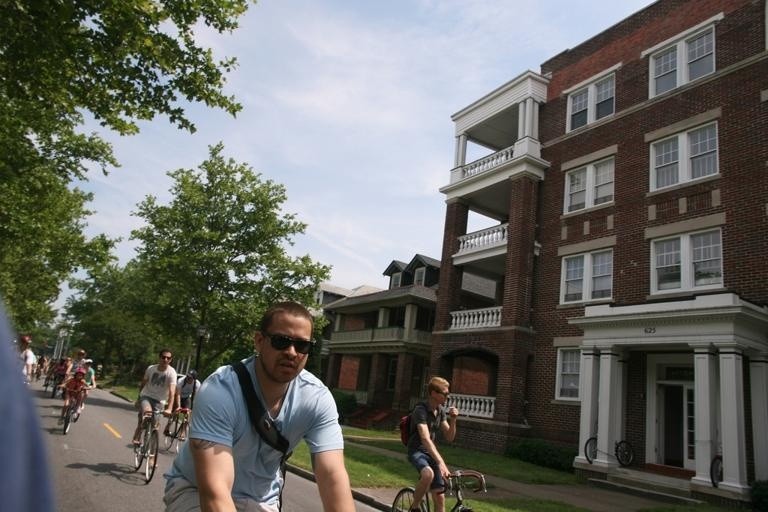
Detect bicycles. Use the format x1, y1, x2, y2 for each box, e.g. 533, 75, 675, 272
391, 469, 490, 512
163, 406, 192, 457
18, 363, 91, 436
132, 408, 170, 483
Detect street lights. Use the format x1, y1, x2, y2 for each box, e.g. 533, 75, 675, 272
194, 323, 209, 370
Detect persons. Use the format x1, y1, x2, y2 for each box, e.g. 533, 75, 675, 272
133, 348, 202, 470
17, 335, 97, 427
0, 298, 56, 512
404, 377, 459, 512
164, 301, 356, 512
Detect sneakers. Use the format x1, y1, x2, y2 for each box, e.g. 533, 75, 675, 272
408, 506, 421, 512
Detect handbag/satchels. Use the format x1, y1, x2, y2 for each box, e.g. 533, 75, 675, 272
279, 450, 293, 511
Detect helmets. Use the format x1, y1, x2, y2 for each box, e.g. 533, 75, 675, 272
75, 349, 93, 375
20, 336, 32, 344
187, 369, 199, 379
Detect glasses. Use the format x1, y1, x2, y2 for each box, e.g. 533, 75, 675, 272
161, 356, 172, 359
436, 390, 450, 397
261, 331, 313, 354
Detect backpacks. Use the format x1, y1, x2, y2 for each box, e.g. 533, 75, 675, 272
176, 373, 186, 389
400, 402, 416, 446
